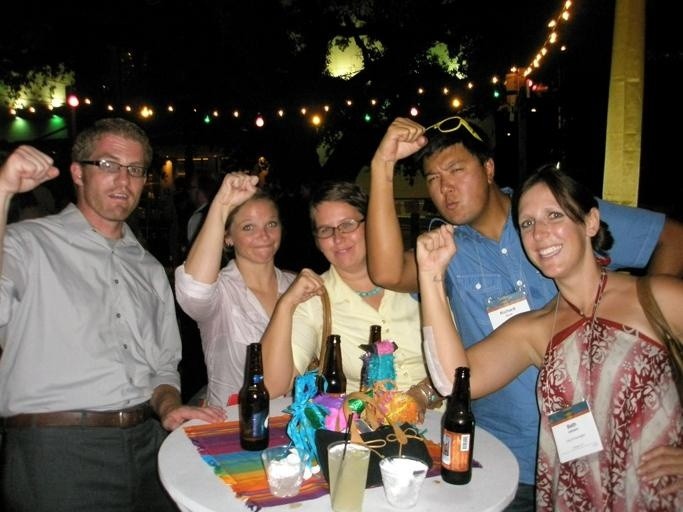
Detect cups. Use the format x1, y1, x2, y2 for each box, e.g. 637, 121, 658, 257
327, 439, 370, 510
379, 457, 427, 505
259, 444, 307, 499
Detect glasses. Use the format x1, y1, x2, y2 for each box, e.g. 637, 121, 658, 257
312, 215, 367, 240
422, 115, 484, 143
80, 159, 149, 181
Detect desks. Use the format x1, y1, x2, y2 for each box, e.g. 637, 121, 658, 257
157, 390, 519, 511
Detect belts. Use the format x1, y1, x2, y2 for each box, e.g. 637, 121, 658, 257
2, 399, 153, 431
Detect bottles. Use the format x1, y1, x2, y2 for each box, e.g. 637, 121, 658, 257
360, 324, 383, 392
440, 365, 481, 487
320, 335, 346, 395
239, 340, 270, 449
